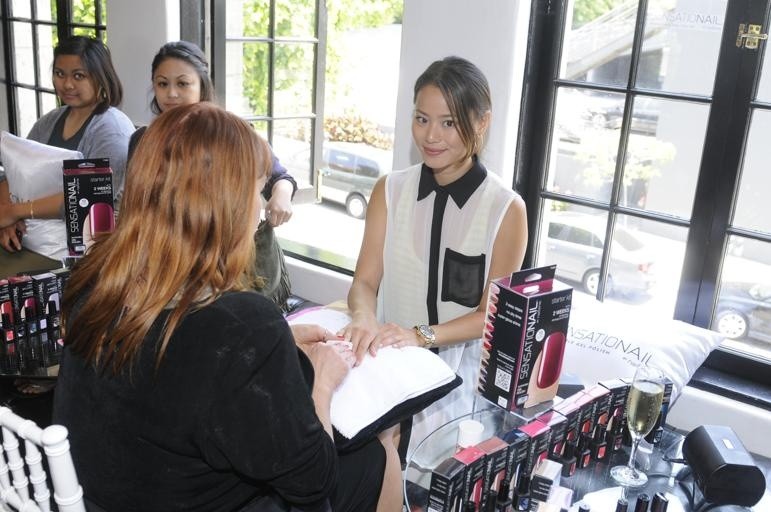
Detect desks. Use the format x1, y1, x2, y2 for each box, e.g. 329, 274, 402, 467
403, 401, 753, 512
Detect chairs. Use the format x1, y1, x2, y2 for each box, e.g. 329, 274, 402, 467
0, 404, 87, 512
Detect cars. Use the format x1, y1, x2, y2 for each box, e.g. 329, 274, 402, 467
545, 212, 661, 299
591, 104, 658, 133
713, 280, 770, 347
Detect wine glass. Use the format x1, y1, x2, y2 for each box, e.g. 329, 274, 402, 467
611, 367, 666, 488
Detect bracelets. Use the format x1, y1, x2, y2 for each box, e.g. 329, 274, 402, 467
29, 198, 36, 220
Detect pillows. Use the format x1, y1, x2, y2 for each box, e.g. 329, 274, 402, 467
0, 130, 83, 262
284, 299, 464, 454
561, 289, 727, 415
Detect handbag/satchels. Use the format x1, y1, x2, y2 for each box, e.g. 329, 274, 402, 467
236, 219, 291, 310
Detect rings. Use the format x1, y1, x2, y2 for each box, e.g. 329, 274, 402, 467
323, 328, 329, 342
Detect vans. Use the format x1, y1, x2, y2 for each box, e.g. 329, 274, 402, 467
312, 144, 390, 219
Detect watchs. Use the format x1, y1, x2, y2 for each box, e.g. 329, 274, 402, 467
412, 323, 437, 351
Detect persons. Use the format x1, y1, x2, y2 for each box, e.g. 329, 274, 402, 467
82, 202, 116, 256
129, 39, 299, 226
46, 97, 410, 512
1, 36, 139, 253
525, 330, 566, 408
338, 54, 530, 428
